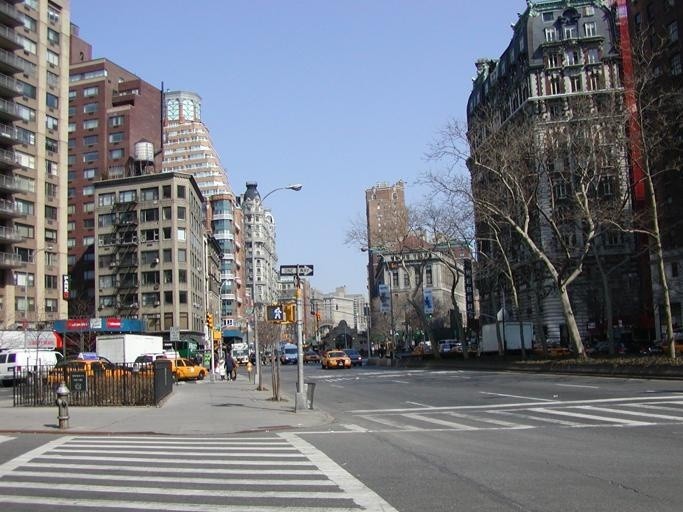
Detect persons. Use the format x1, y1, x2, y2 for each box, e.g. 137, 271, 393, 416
209, 350, 237, 383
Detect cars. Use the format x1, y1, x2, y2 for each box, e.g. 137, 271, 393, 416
46, 351, 207, 383
229, 343, 362, 369
534, 338, 683, 356
410, 338, 471, 355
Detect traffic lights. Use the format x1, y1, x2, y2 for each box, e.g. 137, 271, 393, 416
206, 312, 213, 328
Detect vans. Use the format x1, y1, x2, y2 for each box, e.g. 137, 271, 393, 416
0, 349, 63, 386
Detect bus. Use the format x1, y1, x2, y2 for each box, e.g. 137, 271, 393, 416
162, 338, 197, 358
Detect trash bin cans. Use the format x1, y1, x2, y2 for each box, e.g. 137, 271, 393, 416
296, 382, 315, 409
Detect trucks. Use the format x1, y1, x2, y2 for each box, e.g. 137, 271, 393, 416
95, 334, 181, 367
472, 321, 535, 356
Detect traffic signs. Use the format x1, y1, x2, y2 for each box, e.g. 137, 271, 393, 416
280, 265, 314, 277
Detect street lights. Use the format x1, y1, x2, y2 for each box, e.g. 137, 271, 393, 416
251, 184, 301, 385
360, 247, 394, 359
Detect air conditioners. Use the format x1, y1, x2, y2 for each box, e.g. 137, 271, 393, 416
154, 301, 159, 306
152, 258, 159, 264
